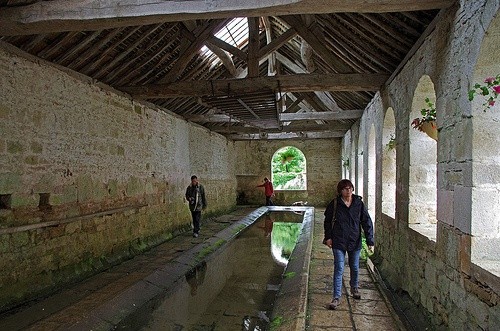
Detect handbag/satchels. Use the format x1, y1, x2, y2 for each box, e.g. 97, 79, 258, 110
322, 222, 334, 246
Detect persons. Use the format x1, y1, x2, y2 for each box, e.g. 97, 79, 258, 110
324, 179, 374, 309
186, 175, 206, 237
185, 260, 207, 295
258, 214, 273, 237
257, 177, 274, 206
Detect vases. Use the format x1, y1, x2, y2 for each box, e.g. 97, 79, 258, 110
422, 120, 438, 140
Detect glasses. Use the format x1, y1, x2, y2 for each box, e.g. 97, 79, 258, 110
343, 187, 353, 190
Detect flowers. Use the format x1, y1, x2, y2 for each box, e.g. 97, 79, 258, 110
468, 74, 500, 111
410, 97, 436, 131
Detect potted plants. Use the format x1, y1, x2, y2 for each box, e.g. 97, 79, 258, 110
281, 152, 295, 163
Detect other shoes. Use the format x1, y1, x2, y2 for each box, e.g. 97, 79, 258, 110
192, 230, 200, 238
350, 286, 362, 299
329, 297, 340, 310
